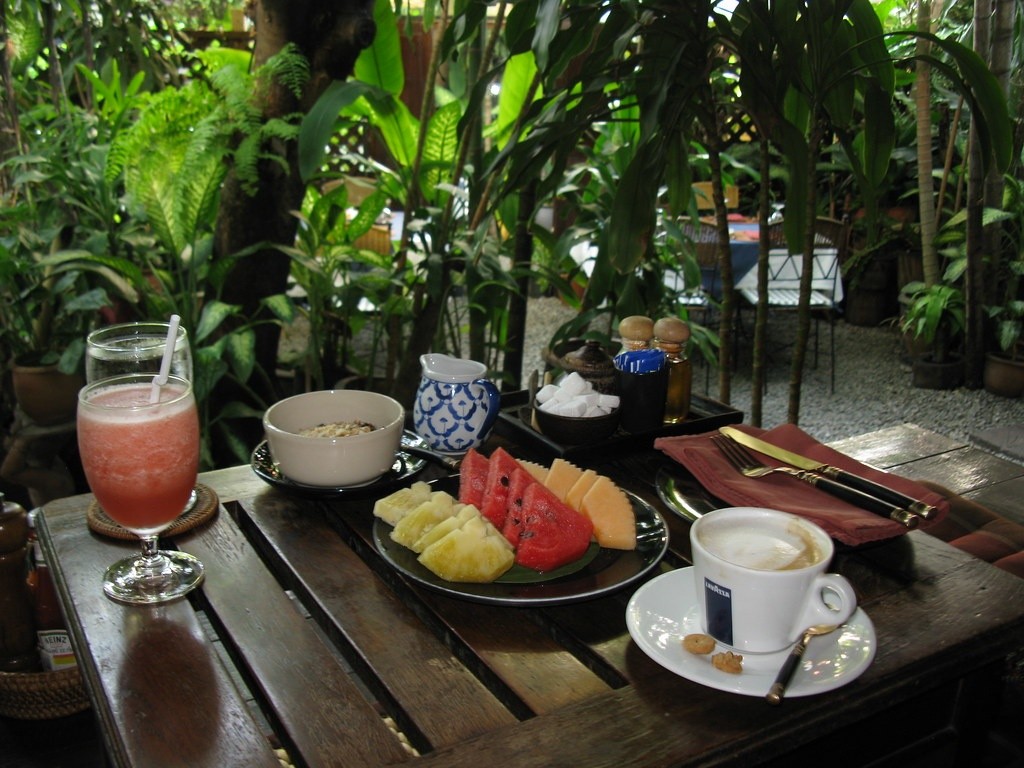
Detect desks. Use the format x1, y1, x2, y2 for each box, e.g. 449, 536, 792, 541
286, 212, 451, 310
570, 226, 843, 376
31, 437, 1024, 767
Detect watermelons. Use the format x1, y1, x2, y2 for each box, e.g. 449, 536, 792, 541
458, 446, 593, 571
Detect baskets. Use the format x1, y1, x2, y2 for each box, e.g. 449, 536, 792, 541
0, 666, 92, 719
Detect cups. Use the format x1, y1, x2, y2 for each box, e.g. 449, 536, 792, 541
690, 508, 856, 654
87, 321, 197, 514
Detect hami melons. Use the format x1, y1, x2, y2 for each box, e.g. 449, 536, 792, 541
516, 456, 636, 551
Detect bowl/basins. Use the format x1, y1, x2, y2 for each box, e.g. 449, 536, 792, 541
534, 398, 618, 446
263, 391, 405, 486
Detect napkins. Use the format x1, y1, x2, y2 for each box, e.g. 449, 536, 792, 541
654, 421, 955, 549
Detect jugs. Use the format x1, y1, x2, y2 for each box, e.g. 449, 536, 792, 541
414, 353, 501, 454
549, 339, 614, 395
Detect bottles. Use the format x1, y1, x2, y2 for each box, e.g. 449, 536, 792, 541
615, 316, 694, 424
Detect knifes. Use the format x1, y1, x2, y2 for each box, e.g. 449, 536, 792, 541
719, 425, 936, 519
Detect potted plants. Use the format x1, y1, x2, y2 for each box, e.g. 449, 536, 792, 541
1, 246, 81, 425
976, 206, 1023, 398
889, 275, 965, 393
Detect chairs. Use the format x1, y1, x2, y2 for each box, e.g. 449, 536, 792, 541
323, 176, 391, 209
656, 179, 849, 396
313, 220, 390, 257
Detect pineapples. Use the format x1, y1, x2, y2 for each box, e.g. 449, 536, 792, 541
373, 480, 516, 583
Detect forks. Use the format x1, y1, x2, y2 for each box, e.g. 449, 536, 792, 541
710, 433, 918, 534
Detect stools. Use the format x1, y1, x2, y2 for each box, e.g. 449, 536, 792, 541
906, 481, 1024, 577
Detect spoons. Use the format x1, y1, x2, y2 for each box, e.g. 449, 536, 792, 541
765, 625, 837, 707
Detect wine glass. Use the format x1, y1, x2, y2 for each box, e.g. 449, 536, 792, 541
78, 375, 206, 605
771, 204, 784, 220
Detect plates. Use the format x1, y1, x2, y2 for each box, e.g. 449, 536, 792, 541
626, 565, 876, 697
251, 431, 430, 493
375, 474, 670, 604
